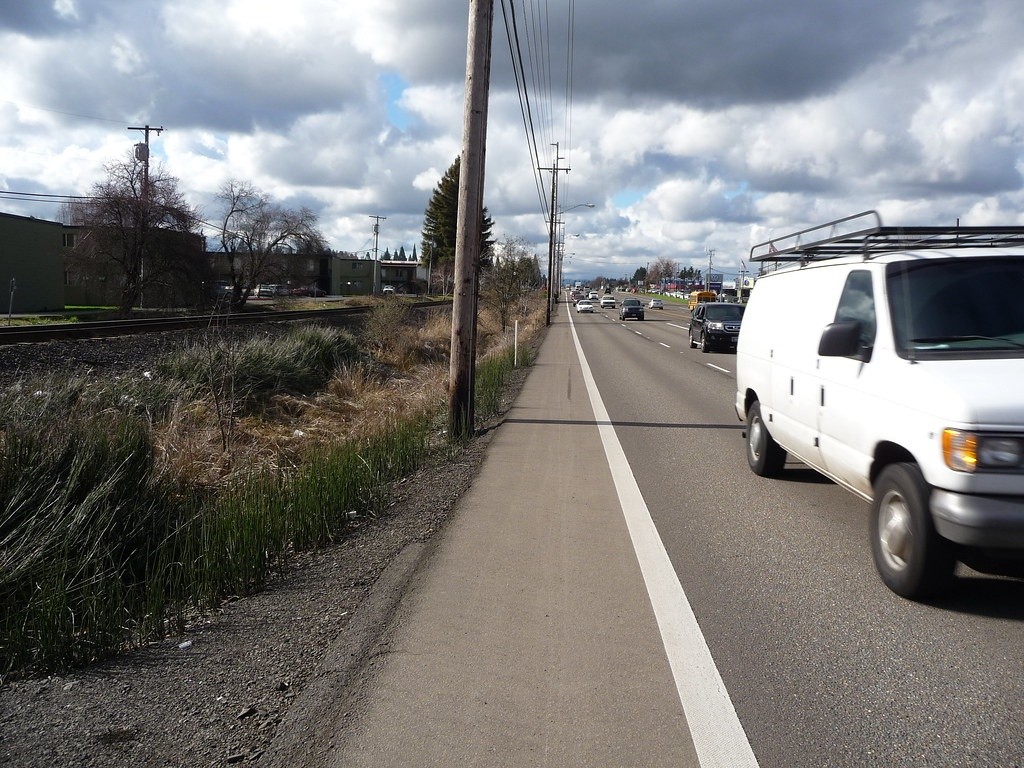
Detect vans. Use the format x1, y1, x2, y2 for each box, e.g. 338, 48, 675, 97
215, 280, 234, 298
604, 288, 611, 294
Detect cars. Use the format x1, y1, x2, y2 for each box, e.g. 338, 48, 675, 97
570, 290, 580, 299
638, 289, 660, 294
254, 285, 272, 297
583, 287, 591, 296
689, 300, 746, 354
576, 300, 594, 313
717, 294, 749, 303
383, 285, 395, 294
648, 299, 663, 310
293, 285, 327, 297
663, 291, 689, 299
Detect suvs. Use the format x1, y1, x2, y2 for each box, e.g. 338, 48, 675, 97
736, 209, 1024, 598
589, 291, 599, 300
573, 294, 585, 306
620, 297, 645, 321
600, 295, 616, 309
269, 284, 291, 296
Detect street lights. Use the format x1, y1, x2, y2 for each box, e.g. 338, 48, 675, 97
551, 203, 595, 313
557, 234, 579, 299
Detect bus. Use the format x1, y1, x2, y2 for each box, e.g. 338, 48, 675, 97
688, 291, 716, 313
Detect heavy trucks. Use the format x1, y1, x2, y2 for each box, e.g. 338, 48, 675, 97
575, 282, 581, 290
